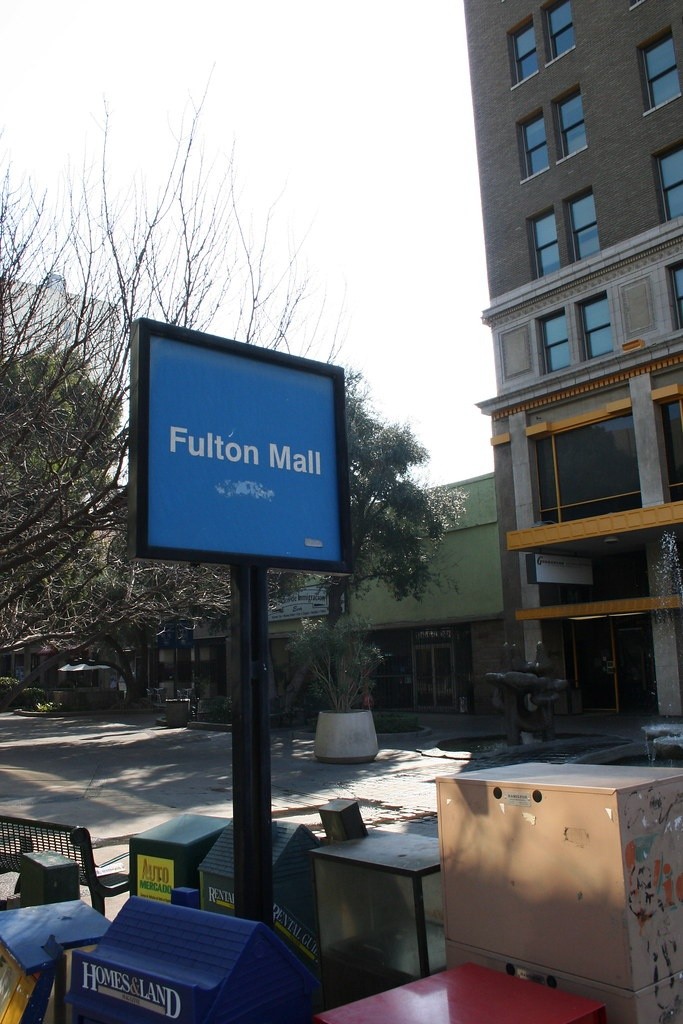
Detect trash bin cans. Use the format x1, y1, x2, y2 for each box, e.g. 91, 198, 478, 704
166, 698, 190, 728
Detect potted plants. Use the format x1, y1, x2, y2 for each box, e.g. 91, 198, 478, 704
292, 614, 383, 763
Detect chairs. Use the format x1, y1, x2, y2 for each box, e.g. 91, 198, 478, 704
0, 813, 145, 919
